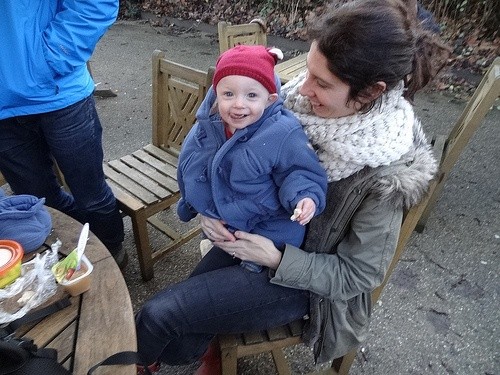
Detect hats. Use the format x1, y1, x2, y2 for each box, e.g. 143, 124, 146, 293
212, 45, 283, 97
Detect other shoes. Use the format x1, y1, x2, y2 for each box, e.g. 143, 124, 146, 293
115, 243, 129, 277
136, 361, 160, 375
192, 344, 222, 375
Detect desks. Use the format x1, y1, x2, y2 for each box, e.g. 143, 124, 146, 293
274, 52, 307, 84
0, 205, 138, 375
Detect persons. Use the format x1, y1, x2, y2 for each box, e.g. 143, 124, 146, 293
177, 45, 326, 273
134, 1, 446, 375
0, 1, 129, 272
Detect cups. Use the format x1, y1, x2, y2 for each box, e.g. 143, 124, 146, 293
52, 254, 94, 297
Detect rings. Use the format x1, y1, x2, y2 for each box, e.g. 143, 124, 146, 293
232, 254, 235, 259
209, 230, 213, 235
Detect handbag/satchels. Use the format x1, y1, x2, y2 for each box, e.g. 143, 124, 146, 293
0, 292, 144, 375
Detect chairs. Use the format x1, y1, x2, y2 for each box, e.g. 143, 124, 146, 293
402, 56, 500, 234
218, 22, 267, 56
200, 179, 437, 375
103, 50, 217, 282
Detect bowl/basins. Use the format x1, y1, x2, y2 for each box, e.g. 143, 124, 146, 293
0, 240, 24, 290
